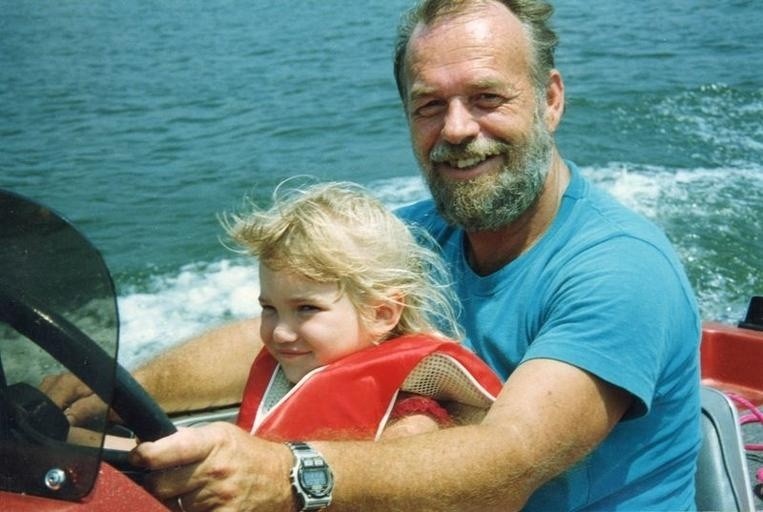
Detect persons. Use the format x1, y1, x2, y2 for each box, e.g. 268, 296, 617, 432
213, 171, 504, 447
36, 0, 708, 512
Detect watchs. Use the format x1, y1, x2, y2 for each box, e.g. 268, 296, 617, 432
282, 440, 336, 512
